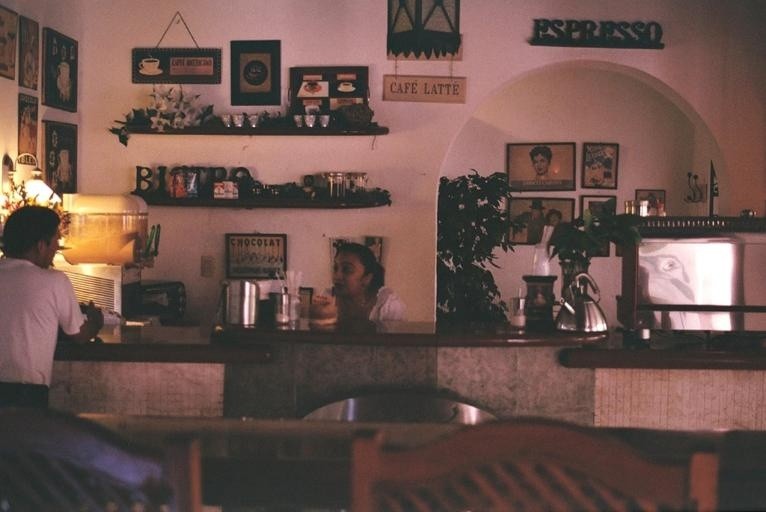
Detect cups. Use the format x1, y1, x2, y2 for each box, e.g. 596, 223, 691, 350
295, 115, 329, 128
304, 105, 319, 115
624, 200, 666, 217
511, 297, 525, 329
138, 58, 159, 73
271, 293, 290, 325
340, 82, 352, 90
222, 114, 258, 128
290, 295, 301, 322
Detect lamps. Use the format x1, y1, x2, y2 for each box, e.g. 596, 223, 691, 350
10, 152, 62, 204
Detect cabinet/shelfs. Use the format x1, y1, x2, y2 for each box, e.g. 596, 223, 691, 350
117, 122, 391, 208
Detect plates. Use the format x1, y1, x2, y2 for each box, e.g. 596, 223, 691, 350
139, 69, 163, 76
337, 86, 356, 93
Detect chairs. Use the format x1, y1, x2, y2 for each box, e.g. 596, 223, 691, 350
349, 417, 721, 512
0, 404, 222, 512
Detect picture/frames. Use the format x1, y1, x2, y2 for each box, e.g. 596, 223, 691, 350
506, 197, 575, 245
581, 142, 619, 189
506, 142, 576, 191
635, 189, 665, 216
580, 195, 616, 218
231, 40, 281, 106
224, 232, 287, 280
0, 4, 78, 196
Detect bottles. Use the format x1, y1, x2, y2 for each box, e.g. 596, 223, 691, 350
326, 172, 367, 200
534, 245, 550, 276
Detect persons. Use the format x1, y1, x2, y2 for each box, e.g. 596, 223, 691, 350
514, 199, 561, 243
0, 205, 104, 409
322, 243, 407, 320
529, 147, 560, 179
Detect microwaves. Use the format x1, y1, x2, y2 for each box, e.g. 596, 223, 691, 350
122, 280, 187, 322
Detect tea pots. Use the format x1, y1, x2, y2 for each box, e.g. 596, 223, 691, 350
554, 273, 608, 332
